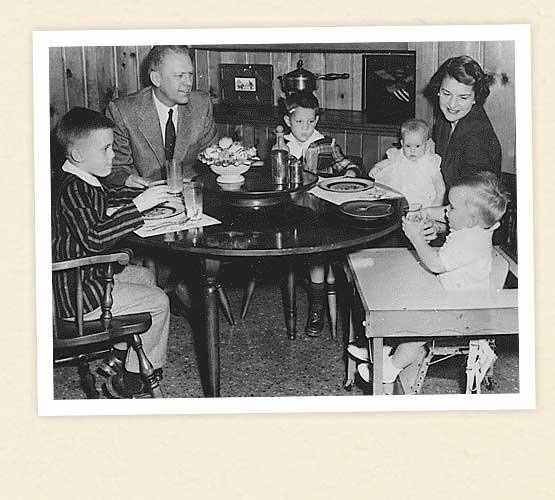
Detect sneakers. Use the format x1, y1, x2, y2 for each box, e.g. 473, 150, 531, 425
358, 363, 396, 383
347, 345, 369, 361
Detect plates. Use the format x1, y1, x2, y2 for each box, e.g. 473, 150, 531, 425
338, 200, 394, 219
317, 177, 376, 193
144, 201, 185, 219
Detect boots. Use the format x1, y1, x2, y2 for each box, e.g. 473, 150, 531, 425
305, 286, 326, 337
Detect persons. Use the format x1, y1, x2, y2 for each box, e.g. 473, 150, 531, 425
345, 166, 511, 384
420, 55, 503, 207
271, 91, 363, 337
104, 46, 220, 314
368, 119, 447, 233
52, 106, 185, 399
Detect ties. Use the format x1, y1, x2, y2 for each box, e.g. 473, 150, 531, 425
165, 109, 176, 160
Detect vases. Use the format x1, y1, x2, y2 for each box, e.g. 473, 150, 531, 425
209, 165, 250, 183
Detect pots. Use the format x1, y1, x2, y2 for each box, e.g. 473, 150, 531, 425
280, 58, 351, 93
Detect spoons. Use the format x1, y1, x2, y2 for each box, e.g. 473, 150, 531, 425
355, 202, 392, 213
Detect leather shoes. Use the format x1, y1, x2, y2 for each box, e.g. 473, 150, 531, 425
105, 373, 152, 398
99, 352, 123, 375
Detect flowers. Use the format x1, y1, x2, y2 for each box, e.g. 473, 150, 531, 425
196, 137, 259, 167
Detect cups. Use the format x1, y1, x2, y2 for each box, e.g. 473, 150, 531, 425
180, 182, 205, 221
402, 203, 423, 223
166, 160, 184, 196
287, 159, 304, 185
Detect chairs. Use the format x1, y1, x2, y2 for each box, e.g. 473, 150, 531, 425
51, 247, 162, 398
395, 247, 511, 395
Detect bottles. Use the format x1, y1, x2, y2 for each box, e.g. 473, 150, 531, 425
271, 125, 290, 185
